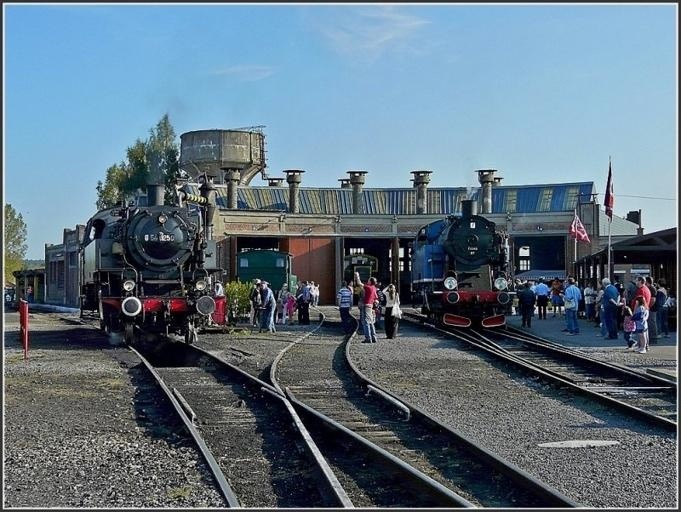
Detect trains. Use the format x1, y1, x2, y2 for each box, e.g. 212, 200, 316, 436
344, 254, 379, 301
79, 178, 224, 344
411, 200, 516, 332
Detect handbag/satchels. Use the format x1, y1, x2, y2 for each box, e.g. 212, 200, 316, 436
391, 292, 403, 320
565, 299, 576, 309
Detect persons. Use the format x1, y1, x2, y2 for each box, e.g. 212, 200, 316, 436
249, 278, 319, 334
337, 272, 384, 343
514, 275, 668, 353
382, 284, 400, 338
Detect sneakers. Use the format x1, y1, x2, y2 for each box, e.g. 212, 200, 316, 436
553, 314, 671, 354
361, 335, 394, 344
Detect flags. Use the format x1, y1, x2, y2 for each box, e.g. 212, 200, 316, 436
626, 211, 639, 225
604, 162, 613, 223
571, 216, 591, 243
